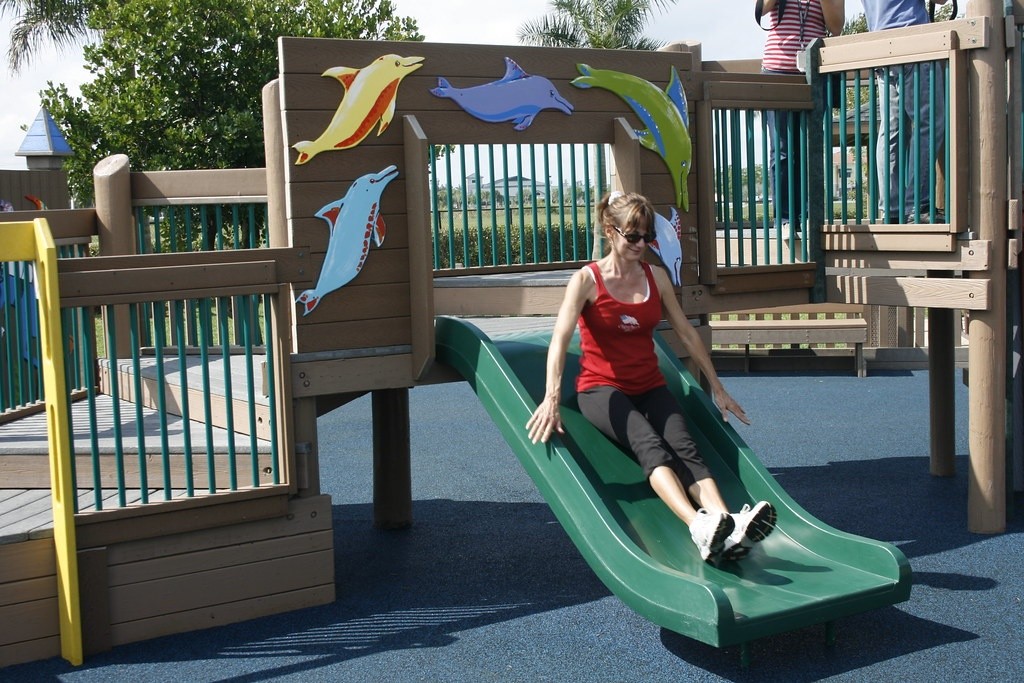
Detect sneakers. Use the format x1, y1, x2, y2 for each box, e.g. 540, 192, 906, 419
881, 208, 946, 225
780, 223, 796, 240
690, 501, 778, 564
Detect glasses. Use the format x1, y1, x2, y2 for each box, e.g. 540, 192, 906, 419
610, 224, 657, 244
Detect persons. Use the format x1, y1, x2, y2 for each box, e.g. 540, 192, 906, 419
932, 0, 1024, 222
523, 191, 777, 570
858, 0, 949, 225
754, 0, 846, 238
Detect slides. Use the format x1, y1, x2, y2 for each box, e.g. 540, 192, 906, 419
435, 308, 914, 651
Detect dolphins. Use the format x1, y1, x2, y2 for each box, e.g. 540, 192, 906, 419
647, 206, 685, 290
431, 54, 575, 132
571, 60, 693, 213
292, 165, 400, 316
289, 53, 426, 165
